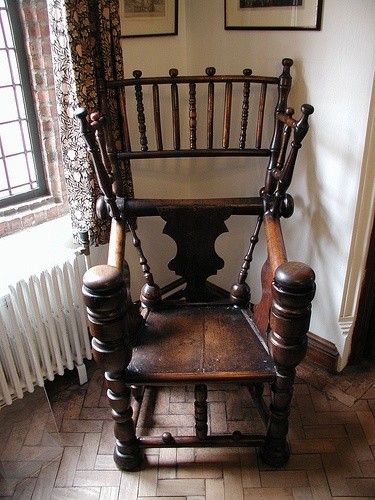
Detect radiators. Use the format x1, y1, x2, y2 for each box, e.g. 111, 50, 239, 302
0, 231, 95, 409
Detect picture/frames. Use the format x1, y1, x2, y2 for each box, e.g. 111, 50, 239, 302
119, 0, 178, 38
224, 0, 324, 31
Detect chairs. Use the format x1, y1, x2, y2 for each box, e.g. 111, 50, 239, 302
71, 53, 317, 471
